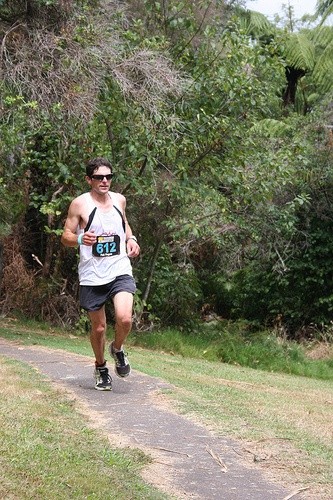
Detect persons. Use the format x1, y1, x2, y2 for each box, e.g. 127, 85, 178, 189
60, 157, 140, 391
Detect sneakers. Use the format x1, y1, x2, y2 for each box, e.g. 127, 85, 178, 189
107, 342, 130, 378
93, 360, 113, 391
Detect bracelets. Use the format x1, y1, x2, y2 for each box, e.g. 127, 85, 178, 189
77, 233, 84, 245
125, 236, 137, 242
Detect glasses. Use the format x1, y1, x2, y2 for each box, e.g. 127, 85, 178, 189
90, 173, 114, 180
